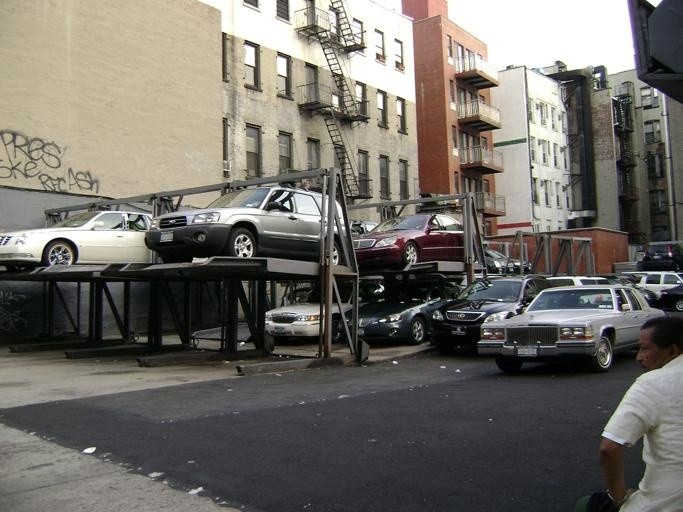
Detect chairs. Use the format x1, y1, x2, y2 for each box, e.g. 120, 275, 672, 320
558, 295, 578, 308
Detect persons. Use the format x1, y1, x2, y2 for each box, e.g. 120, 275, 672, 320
587, 316, 683, 511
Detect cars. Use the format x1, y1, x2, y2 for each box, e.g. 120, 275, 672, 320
483, 246, 534, 275
352, 213, 478, 270
1, 209, 157, 271
636, 242, 682, 272
144, 185, 348, 263
